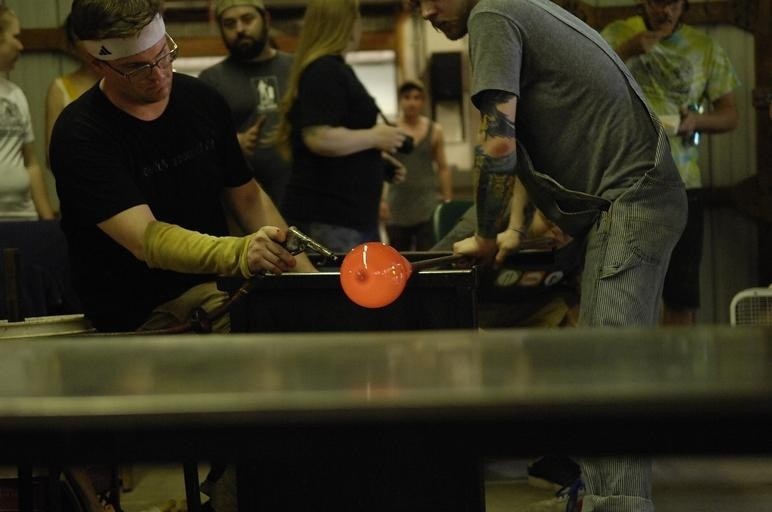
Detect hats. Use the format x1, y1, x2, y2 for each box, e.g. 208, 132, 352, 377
397, 74, 427, 94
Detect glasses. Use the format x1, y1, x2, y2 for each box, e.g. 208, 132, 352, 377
100, 30, 181, 85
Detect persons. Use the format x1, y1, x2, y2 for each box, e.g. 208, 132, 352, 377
45, 12, 103, 172
201, 2, 300, 198
384, 79, 452, 255
419, 2, 690, 510
599, 3, 738, 328
1, 8, 60, 311
286, 1, 408, 257
55, 1, 331, 492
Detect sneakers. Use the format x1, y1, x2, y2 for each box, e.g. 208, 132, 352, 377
525, 485, 594, 512
58, 461, 126, 512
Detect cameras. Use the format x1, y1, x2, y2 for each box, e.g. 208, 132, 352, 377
383, 124, 413, 156
